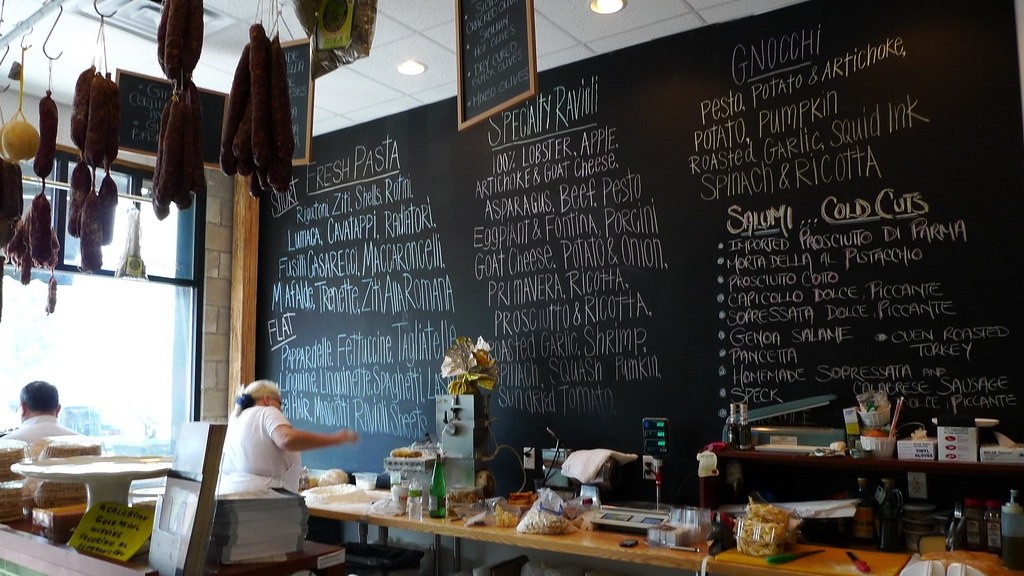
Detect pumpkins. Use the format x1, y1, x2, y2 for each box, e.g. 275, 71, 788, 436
863, 429, 885, 437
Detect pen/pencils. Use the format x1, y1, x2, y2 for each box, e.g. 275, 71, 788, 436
665, 545, 700, 553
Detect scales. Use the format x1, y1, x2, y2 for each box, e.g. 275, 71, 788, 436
590, 418, 672, 535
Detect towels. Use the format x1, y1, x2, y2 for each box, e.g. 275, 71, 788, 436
560, 448, 639, 483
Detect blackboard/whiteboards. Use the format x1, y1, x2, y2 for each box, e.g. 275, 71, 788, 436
453, 0, 539, 133
276, 38, 318, 166
254, 0, 1024, 558
115, 68, 230, 171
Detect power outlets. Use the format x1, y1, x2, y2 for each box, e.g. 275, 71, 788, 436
525, 447, 535, 469
908, 471, 927, 498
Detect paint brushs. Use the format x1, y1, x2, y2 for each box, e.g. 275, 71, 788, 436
889, 396, 904, 437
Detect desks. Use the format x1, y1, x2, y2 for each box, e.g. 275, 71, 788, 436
300, 483, 912, 576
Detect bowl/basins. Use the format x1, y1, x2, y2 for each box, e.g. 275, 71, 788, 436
735, 517, 784, 556
902, 504, 948, 553
857, 403, 891, 427
352, 471, 378, 490
860, 436, 896, 458
447, 485, 484, 518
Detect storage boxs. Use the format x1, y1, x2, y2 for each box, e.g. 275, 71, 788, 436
722, 394, 846, 447
895, 426, 1024, 462
390, 469, 436, 502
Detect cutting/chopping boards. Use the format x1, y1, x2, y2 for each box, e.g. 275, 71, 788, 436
714, 543, 912, 576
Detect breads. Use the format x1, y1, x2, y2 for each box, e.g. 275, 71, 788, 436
389, 448, 422, 457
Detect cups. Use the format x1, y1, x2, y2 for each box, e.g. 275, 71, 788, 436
391, 485, 408, 514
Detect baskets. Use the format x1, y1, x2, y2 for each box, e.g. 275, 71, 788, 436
857, 404, 889, 426
860, 435, 897, 457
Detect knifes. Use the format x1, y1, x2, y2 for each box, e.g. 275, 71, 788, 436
846, 552, 870, 573
767, 549, 824, 564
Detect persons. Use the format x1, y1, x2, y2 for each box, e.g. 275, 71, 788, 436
0, 381, 87, 445
219, 379, 358, 537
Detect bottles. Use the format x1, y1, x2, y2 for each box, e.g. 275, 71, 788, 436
737, 404, 751, 450
880, 478, 899, 551
408, 477, 423, 520
727, 403, 738, 450
983, 500, 1002, 555
999, 491, 1024, 569
854, 479, 873, 545
428, 453, 446, 518
962, 498, 984, 550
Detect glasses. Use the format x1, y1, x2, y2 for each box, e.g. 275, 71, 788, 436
270, 398, 286, 412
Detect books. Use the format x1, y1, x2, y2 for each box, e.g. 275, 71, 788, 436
150, 420, 345, 576
